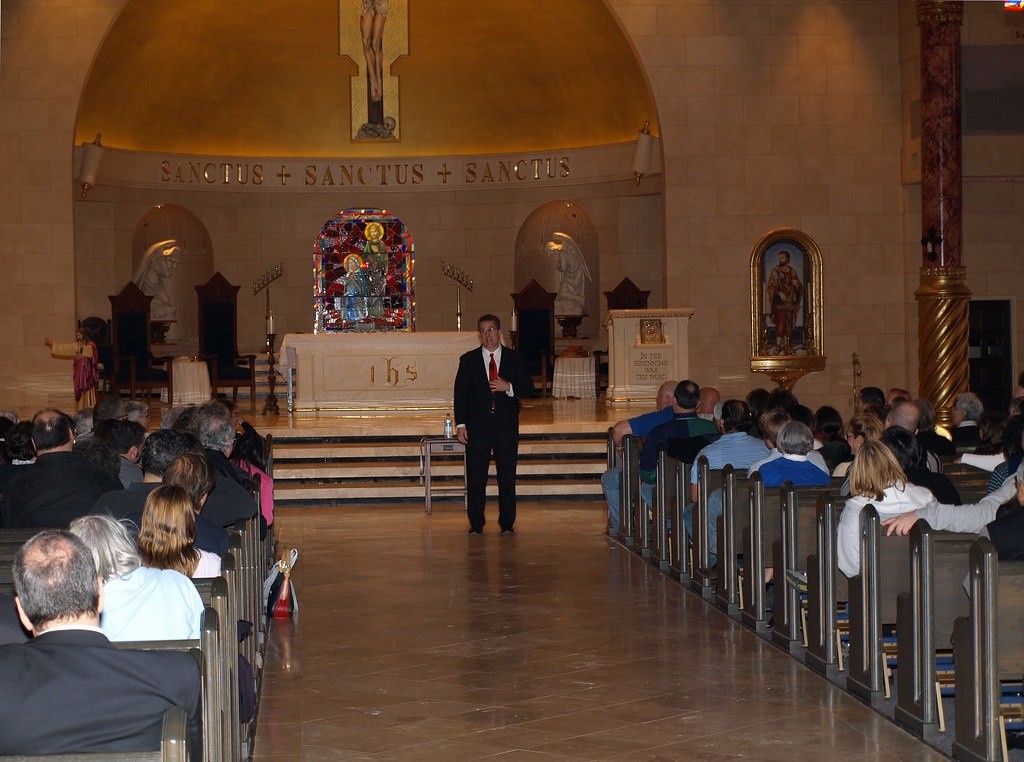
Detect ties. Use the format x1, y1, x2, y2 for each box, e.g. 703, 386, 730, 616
489, 353, 498, 408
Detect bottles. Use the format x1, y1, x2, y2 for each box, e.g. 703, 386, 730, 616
443, 413, 453, 439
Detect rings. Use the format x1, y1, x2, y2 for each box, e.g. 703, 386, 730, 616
494, 385, 496, 388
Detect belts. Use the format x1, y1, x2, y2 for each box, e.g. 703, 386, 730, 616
490, 410, 497, 414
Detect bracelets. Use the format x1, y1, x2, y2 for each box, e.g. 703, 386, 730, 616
617, 446, 622, 447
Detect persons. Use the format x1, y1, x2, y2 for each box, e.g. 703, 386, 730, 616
360, 0, 388, 102
0, 395, 273, 762
136, 240, 180, 318
45, 328, 98, 412
602, 371, 1024, 610
544, 232, 591, 313
454, 314, 533, 534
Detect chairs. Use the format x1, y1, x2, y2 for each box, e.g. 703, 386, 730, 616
507, 278, 558, 398
192, 272, 257, 403
108, 280, 176, 408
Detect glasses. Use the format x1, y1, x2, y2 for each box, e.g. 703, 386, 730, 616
480, 327, 499, 334
116, 414, 128, 420
844, 434, 856, 439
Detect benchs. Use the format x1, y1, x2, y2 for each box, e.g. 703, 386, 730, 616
0, 432, 274, 762
605, 427, 1024, 762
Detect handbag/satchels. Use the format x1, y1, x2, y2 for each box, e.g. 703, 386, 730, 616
273, 545, 292, 621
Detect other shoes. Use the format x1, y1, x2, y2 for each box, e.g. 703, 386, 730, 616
697, 565, 718, 579
468, 526, 482, 535
501, 525, 514, 534
